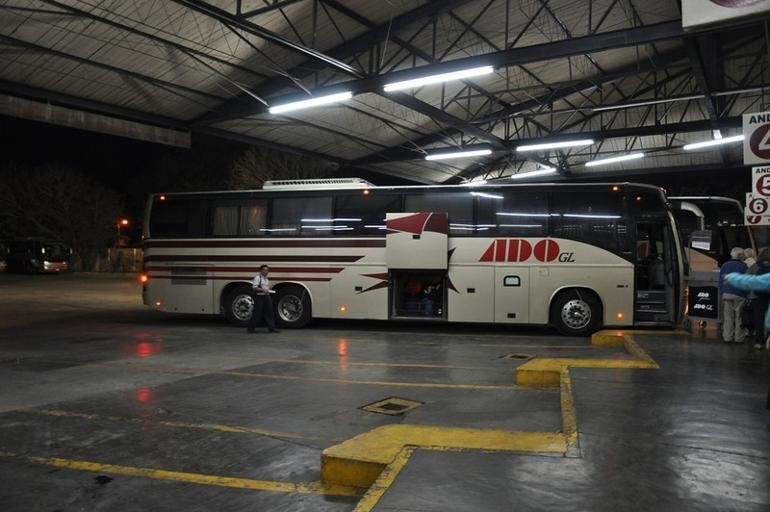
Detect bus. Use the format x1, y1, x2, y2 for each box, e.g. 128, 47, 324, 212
0, 237, 73, 274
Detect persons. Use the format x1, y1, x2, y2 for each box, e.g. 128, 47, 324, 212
247, 265, 280, 334
718, 247, 770, 348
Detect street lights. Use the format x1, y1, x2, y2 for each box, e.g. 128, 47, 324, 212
116, 219, 129, 245
